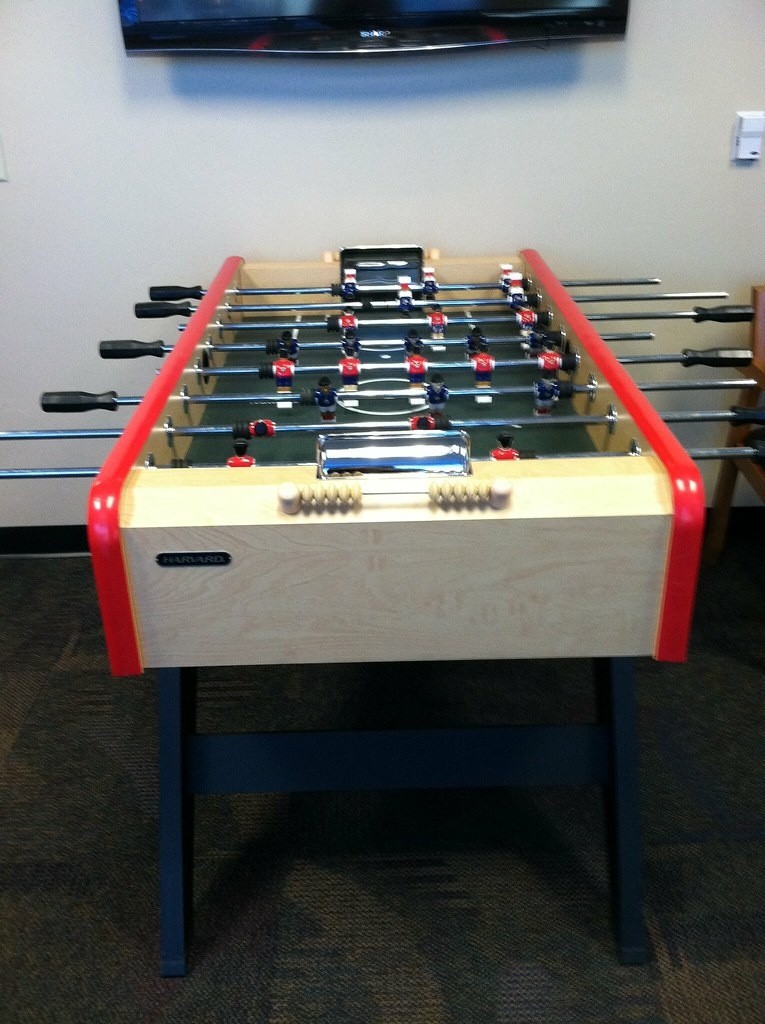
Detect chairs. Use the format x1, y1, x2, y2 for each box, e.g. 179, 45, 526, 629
706, 285, 765, 555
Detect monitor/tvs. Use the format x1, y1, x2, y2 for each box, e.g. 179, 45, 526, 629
118, 0, 631, 61
